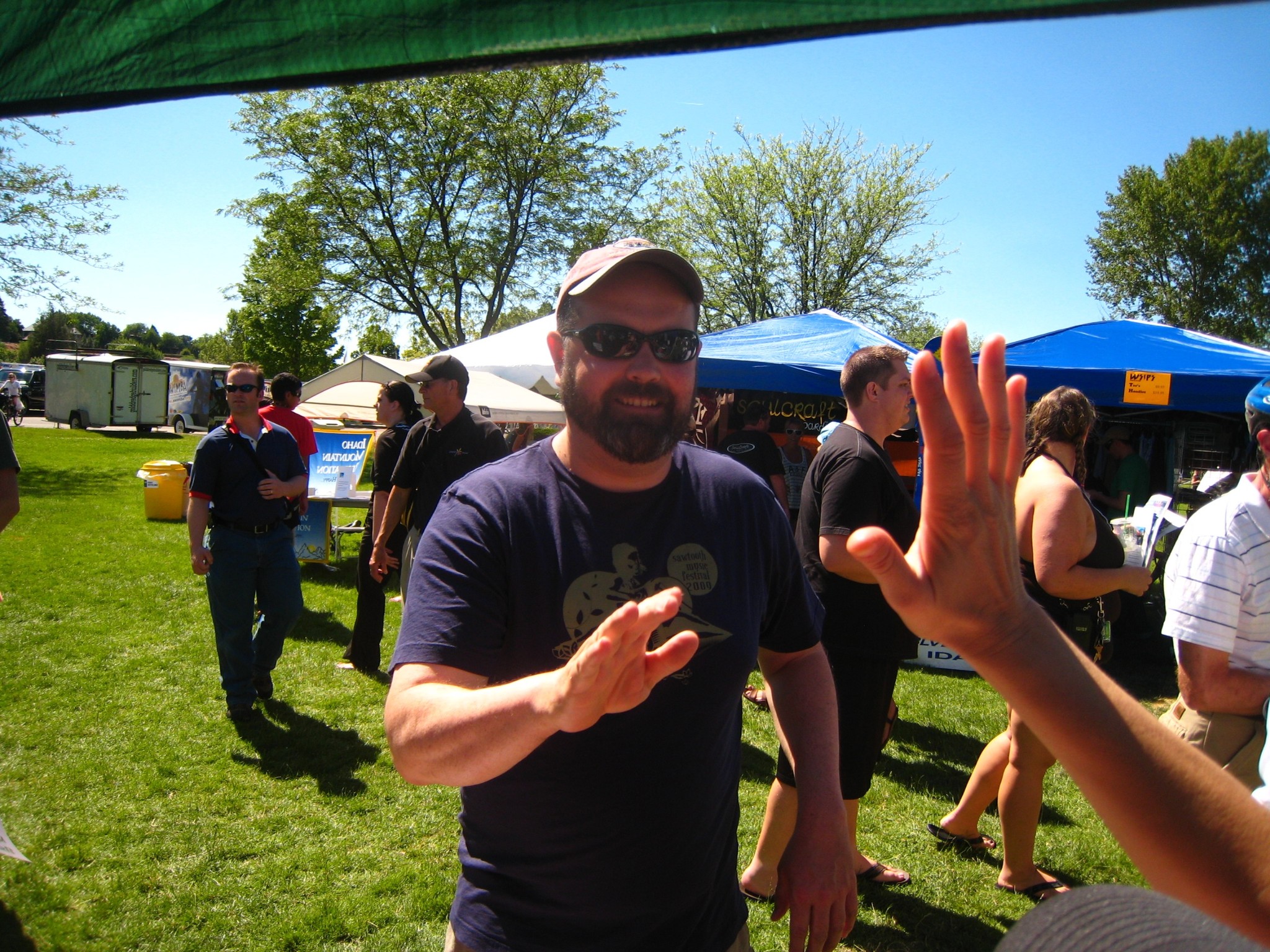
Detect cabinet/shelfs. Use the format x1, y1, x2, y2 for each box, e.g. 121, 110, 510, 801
1159, 419, 1256, 608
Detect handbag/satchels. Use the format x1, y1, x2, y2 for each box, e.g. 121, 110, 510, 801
1108, 591, 1180, 699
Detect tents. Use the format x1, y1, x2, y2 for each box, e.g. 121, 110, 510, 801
412, 307, 562, 399
295, 347, 569, 426
971, 318, 1270, 412
695, 307, 945, 401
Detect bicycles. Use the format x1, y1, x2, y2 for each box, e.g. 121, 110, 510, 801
0, 392, 24, 426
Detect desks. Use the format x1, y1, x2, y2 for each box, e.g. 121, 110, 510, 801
308, 497, 372, 508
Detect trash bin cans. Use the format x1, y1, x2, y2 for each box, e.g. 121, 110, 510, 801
140, 459, 187, 519
180, 461, 193, 517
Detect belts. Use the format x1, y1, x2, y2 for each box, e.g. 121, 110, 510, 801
219, 525, 275, 534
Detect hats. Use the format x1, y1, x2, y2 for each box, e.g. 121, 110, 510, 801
404, 355, 469, 386
556, 236, 703, 335
816, 421, 841, 445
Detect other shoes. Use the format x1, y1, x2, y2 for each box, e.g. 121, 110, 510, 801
253, 670, 273, 698
338, 661, 379, 672
226, 696, 252, 717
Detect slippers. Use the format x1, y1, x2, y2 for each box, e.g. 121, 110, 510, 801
738, 880, 774, 904
994, 871, 1066, 900
742, 686, 769, 705
856, 857, 910, 885
881, 707, 900, 747
927, 821, 997, 849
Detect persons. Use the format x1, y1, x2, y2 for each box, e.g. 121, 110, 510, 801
1157, 375, 1270, 794
840, 324, 1270, 952
366, 354, 510, 610
0, 410, 23, 604
1084, 424, 1151, 521
333, 380, 426, 676
921, 383, 1154, 901
717, 403, 849, 536
0, 373, 23, 416
730, 345, 914, 900
384, 234, 862, 952
256, 370, 319, 639
186, 364, 309, 723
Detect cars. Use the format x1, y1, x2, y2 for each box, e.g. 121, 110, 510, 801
0, 362, 46, 417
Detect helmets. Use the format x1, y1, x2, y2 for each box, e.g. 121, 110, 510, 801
1245, 376, 1270, 435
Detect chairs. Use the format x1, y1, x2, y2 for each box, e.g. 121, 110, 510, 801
336, 508, 370, 565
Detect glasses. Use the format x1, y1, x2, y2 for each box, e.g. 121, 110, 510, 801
562, 323, 700, 363
291, 391, 301, 398
224, 384, 259, 393
785, 429, 803, 437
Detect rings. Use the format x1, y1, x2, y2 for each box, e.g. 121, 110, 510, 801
271, 490, 273, 494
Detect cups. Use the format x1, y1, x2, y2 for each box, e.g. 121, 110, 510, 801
1109, 517, 1138, 552
308, 488, 315, 495
